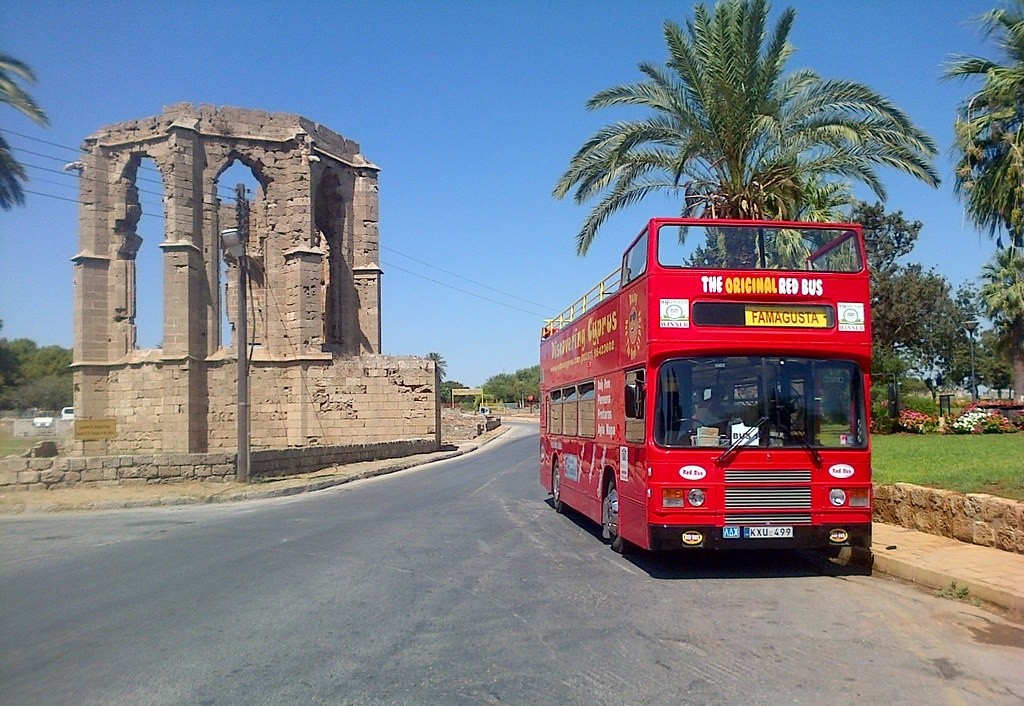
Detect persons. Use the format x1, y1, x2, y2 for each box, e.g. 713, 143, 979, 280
693, 385, 731, 434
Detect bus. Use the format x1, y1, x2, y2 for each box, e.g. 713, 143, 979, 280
539, 216, 902, 560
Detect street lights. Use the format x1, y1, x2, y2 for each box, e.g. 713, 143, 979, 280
963, 320, 981, 401
